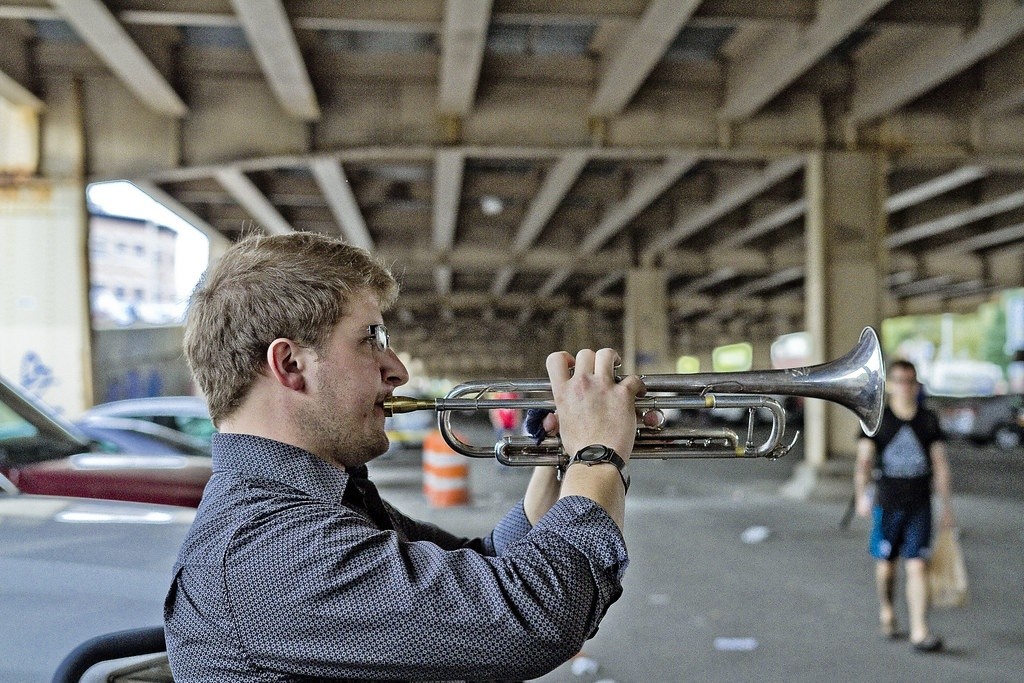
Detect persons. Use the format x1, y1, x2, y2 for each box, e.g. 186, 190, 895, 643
162, 229, 648, 682
852, 359, 954, 652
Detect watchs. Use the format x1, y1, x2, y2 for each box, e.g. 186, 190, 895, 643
564, 443, 631, 495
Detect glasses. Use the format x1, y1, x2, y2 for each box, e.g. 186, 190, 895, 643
317, 323, 390, 353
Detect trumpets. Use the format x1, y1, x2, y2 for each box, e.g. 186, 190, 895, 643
379, 325, 888, 467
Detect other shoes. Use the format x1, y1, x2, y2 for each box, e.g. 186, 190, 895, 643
881, 619, 897, 639
915, 635, 943, 652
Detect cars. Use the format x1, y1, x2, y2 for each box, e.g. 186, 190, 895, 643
0, 375, 228, 683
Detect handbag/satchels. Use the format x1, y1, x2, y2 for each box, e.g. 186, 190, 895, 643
927, 527, 970, 610
